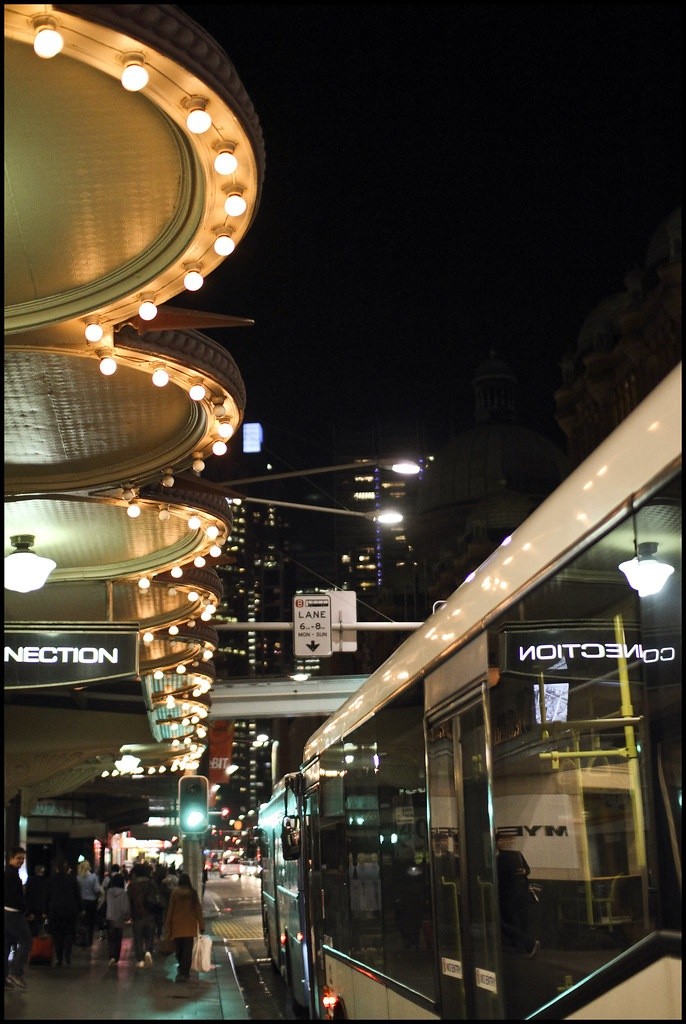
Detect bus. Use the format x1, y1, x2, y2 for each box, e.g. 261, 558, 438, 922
247, 772, 312, 1013
278, 364, 685, 1020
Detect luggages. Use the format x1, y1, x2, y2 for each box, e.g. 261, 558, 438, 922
29, 916, 54, 969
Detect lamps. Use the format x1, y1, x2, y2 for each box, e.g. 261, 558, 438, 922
84, 314, 103, 342
215, 451, 422, 487
117, 48, 149, 93
150, 360, 169, 387
211, 223, 236, 257
210, 434, 227, 456
4, 535, 57, 594
191, 451, 206, 473
157, 503, 171, 522
187, 375, 206, 402
28, 11, 64, 60
102, 542, 223, 779
160, 468, 175, 488
206, 519, 219, 538
619, 539, 638, 591
212, 137, 238, 176
187, 510, 201, 530
637, 540, 675, 598
210, 394, 228, 417
94, 346, 117, 376
126, 499, 140, 518
221, 181, 248, 217
120, 481, 135, 502
180, 94, 212, 135
136, 290, 159, 322
216, 415, 234, 438
245, 497, 404, 525
181, 260, 204, 292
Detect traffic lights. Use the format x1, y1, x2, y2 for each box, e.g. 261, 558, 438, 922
221, 807, 229, 820
179, 774, 212, 834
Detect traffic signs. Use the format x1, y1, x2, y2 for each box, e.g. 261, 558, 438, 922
294, 595, 335, 658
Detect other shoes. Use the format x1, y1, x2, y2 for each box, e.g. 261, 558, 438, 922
57, 961, 63, 966
62, 950, 71, 963
144, 952, 153, 967
176, 972, 189, 981
4, 983, 14, 991
137, 960, 145, 968
8, 973, 26, 988
108, 959, 116, 966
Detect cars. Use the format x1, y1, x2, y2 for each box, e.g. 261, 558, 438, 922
202, 849, 259, 877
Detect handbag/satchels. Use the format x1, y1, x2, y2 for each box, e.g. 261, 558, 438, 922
143, 878, 165, 911
95, 891, 108, 931
190, 923, 212, 973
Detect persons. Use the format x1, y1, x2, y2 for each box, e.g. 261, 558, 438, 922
27, 855, 205, 984
0, 845, 32, 990
